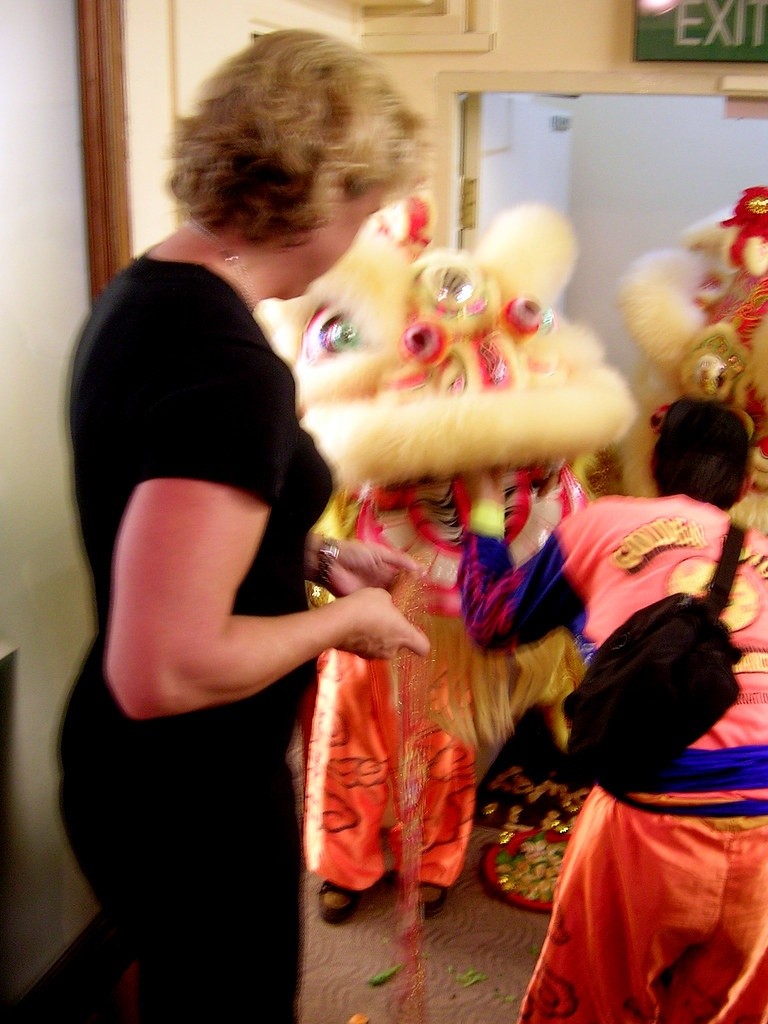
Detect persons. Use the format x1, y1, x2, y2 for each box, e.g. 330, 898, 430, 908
453, 398, 767, 1023
53, 29, 433, 1024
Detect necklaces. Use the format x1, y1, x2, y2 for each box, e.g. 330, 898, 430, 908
189, 217, 258, 306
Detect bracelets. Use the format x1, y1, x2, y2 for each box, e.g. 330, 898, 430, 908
315, 537, 341, 586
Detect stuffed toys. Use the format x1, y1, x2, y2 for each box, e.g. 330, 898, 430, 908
253, 186, 768, 925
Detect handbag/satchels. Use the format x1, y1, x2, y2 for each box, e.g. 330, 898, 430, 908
562, 591, 742, 798
